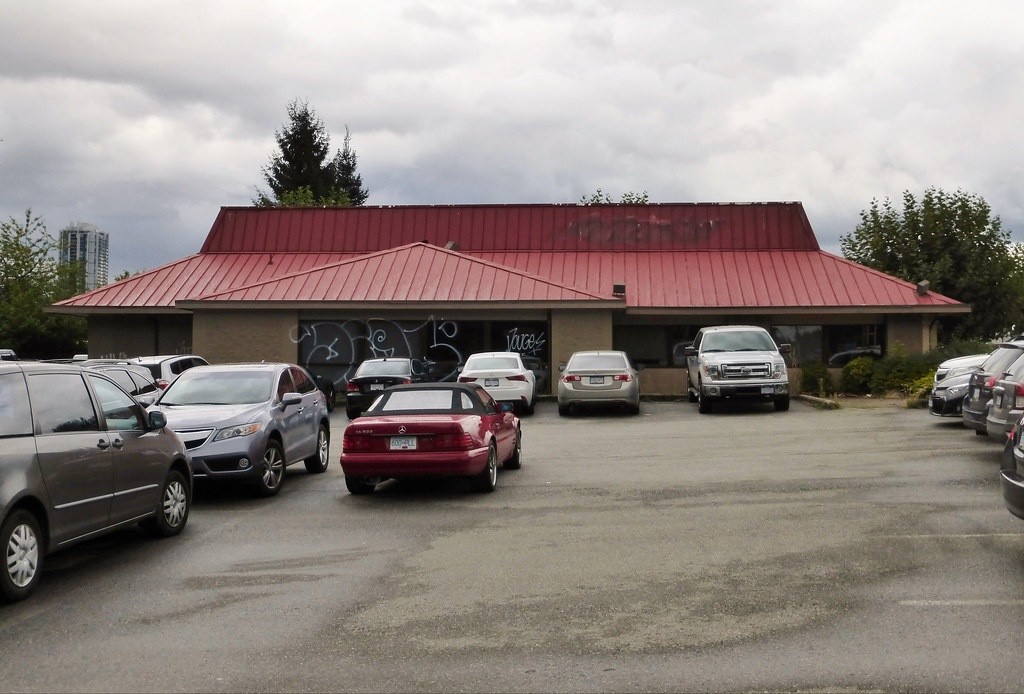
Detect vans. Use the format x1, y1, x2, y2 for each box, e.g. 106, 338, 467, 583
0, 350, 193, 606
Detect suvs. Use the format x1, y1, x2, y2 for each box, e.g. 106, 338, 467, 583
999, 414, 1024, 521
646, 341, 694, 367
928, 346, 999, 418
37, 354, 163, 419
986, 350, 1024, 443
962, 332, 1024, 438
683, 325, 792, 415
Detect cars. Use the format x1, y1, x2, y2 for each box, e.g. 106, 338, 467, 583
343, 356, 434, 419
114, 354, 210, 392
455, 352, 539, 417
521, 357, 551, 394
305, 368, 336, 413
129, 361, 331, 501
557, 349, 644, 416
338, 384, 522, 496
826, 343, 883, 367
